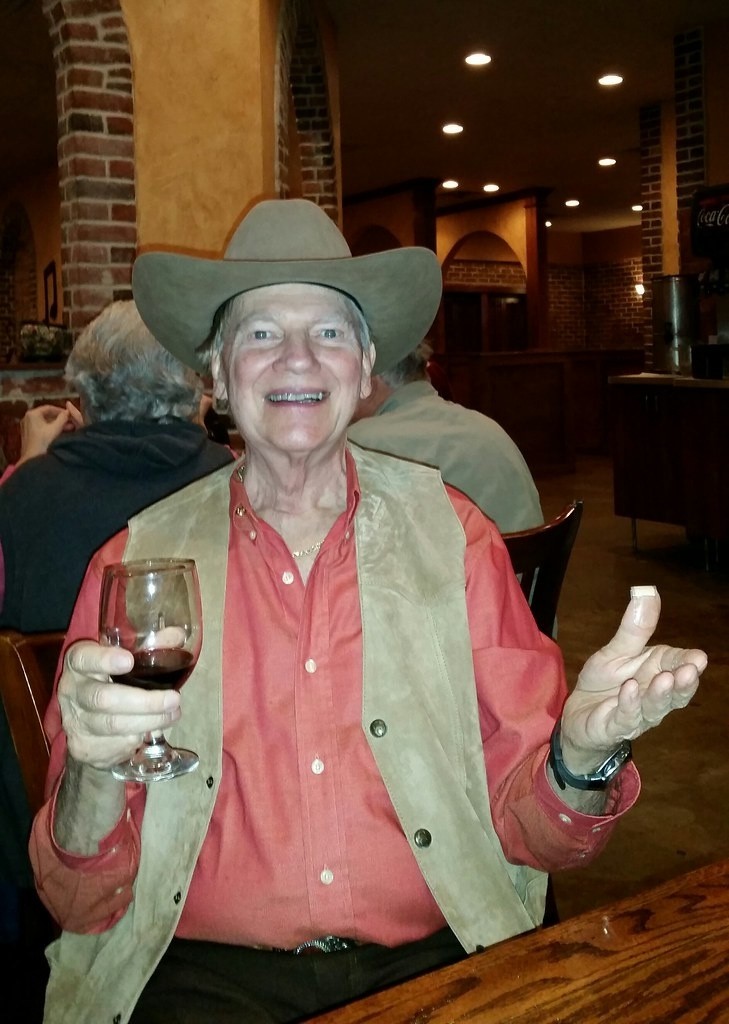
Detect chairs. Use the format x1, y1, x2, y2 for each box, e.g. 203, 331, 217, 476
499, 496, 588, 645
0, 629, 69, 827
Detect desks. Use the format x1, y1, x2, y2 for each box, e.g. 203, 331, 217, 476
291, 855, 729, 1023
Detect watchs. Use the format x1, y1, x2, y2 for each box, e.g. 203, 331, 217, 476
547, 710, 635, 793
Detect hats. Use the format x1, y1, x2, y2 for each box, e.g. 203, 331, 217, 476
131, 201, 441, 378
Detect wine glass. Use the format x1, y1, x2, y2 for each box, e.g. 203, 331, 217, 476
95, 553, 203, 783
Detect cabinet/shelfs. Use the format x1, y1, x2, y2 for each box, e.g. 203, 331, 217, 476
609, 372, 729, 541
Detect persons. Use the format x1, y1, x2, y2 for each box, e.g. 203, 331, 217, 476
1, 296, 256, 647
335, 311, 547, 637
25, 197, 707, 1022
